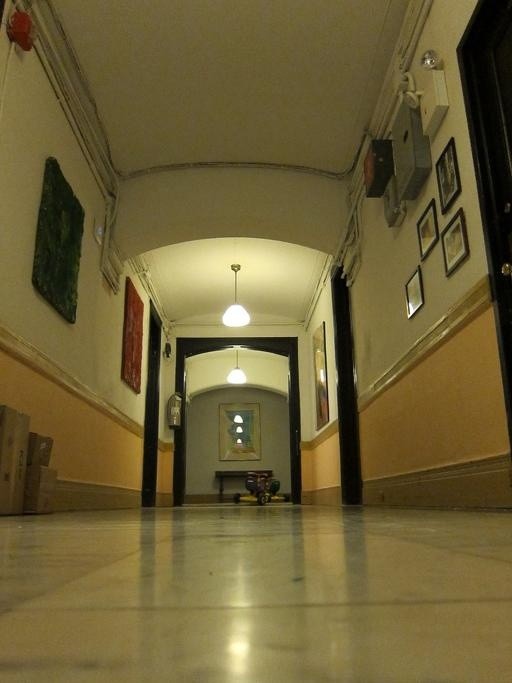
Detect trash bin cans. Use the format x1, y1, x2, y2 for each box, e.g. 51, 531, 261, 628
1, 405, 56, 514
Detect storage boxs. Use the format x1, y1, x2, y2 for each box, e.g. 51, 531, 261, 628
30, 430, 53, 465
25, 465, 58, 514
0, 405, 28, 514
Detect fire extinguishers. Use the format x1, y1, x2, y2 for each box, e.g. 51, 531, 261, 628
167, 392, 183, 426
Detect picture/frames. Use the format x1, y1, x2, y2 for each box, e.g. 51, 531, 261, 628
402, 266, 424, 320
219, 402, 261, 461
440, 208, 469, 279
435, 135, 461, 212
415, 199, 440, 259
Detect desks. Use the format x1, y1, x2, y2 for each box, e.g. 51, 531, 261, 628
216, 469, 272, 501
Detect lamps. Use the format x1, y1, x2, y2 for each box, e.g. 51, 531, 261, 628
220, 265, 250, 327
227, 344, 244, 379
403, 49, 449, 137
